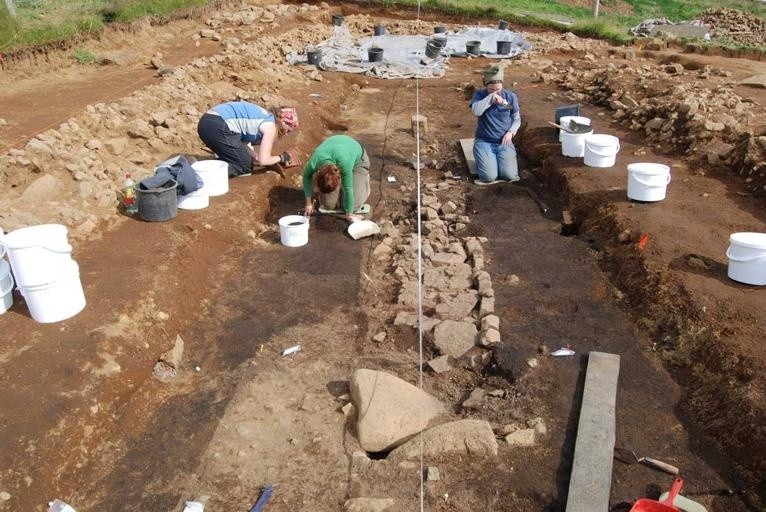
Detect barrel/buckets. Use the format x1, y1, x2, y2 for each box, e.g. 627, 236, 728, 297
135, 178, 178, 220
5, 222, 74, 286
191, 159, 229, 196
0, 228, 6, 262
0, 257, 14, 317
279, 215, 310, 249
17, 262, 87, 324
305, 13, 514, 65
724, 230, 766, 287
178, 173, 211, 210
555, 102, 671, 203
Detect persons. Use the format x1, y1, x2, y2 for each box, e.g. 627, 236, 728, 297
197, 102, 301, 180
468, 64, 522, 181
302, 135, 373, 224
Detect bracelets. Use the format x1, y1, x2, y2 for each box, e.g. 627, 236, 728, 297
507, 130, 514, 138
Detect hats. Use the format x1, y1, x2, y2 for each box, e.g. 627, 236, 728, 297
314, 175, 342, 211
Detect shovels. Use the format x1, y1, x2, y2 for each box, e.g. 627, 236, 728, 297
548, 119, 592, 134
628, 474, 685, 512
614, 447, 678, 474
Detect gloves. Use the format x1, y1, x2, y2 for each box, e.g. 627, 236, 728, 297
279, 151, 290, 163
